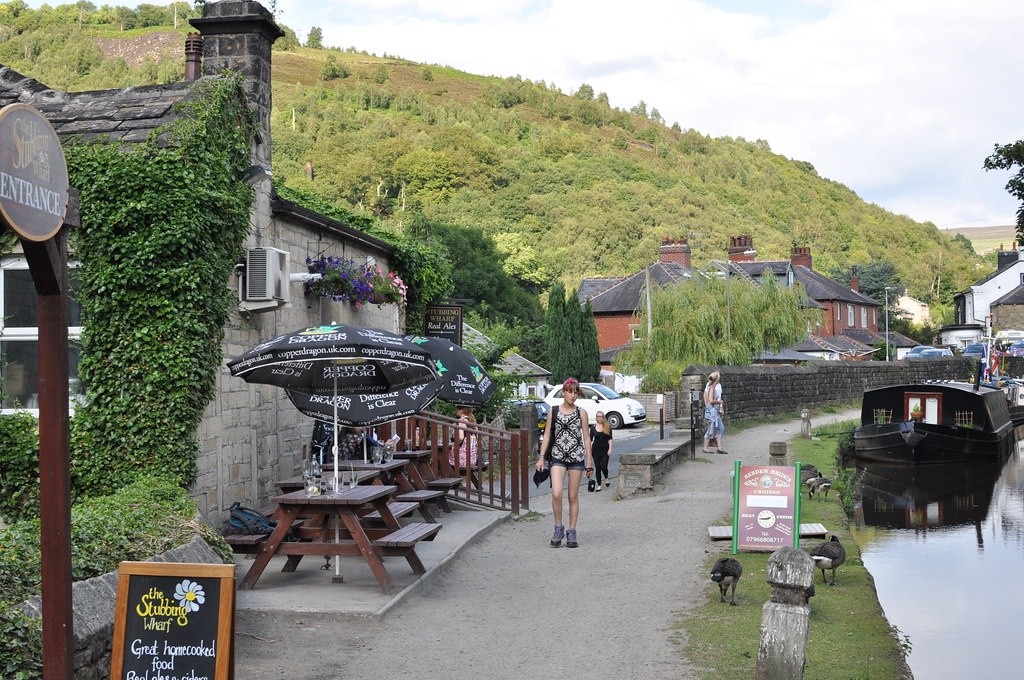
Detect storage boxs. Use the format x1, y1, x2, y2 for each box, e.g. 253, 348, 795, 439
2, 364, 37, 396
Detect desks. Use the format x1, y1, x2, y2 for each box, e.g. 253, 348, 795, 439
320, 459, 442, 527
393, 450, 452, 513
274, 470, 415, 548
238, 485, 427, 594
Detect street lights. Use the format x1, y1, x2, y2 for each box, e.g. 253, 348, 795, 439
726, 250, 756, 366
885, 287, 890, 361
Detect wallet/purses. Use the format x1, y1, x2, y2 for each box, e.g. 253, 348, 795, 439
588, 479, 595, 492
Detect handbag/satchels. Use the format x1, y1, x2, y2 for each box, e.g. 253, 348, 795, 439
476, 459, 487, 471
537, 405, 559, 461
705, 402, 713, 419
719, 404, 724, 416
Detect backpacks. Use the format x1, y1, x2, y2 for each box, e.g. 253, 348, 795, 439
228, 502, 277, 535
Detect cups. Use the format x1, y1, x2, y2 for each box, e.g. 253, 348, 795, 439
348, 473, 358, 489
383, 445, 396, 462
372, 449, 382, 465
404, 439, 412, 453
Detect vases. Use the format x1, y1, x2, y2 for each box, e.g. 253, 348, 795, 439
368, 292, 390, 304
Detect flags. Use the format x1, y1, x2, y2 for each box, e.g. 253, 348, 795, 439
979, 347, 998, 380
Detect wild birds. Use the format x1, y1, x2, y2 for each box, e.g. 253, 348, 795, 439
710, 558, 742, 606
809, 535, 846, 586
808, 478, 831, 502
800, 464, 822, 497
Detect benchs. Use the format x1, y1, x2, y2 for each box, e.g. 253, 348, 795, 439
222, 476, 462, 557
616, 436, 692, 498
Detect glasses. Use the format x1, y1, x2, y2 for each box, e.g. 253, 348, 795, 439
596, 416, 603, 418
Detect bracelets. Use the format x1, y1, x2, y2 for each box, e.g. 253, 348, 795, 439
540, 455, 544, 456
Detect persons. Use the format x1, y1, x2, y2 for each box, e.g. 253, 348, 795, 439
590, 411, 613, 492
702, 371, 727, 454
326, 427, 393, 464
449, 407, 478, 466
534, 378, 592, 548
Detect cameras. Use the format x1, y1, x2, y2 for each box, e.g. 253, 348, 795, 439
588, 479, 596, 492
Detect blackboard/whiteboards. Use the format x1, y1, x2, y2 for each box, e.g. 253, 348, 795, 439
112, 561, 238, 680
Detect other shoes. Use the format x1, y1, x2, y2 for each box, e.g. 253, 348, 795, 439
595, 490, 600, 492
702, 448, 713, 453
606, 483, 609, 487
709, 438, 717, 446
550, 525, 564, 547
716, 449, 727, 454
566, 528, 578, 548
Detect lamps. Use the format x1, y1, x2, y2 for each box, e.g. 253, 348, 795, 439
242, 166, 272, 187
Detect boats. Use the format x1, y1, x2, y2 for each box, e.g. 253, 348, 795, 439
853, 461, 1011, 548
853, 315, 1024, 464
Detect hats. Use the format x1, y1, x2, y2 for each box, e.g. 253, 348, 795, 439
533, 469, 549, 489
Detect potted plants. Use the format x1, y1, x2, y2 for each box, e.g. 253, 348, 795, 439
910, 403, 924, 422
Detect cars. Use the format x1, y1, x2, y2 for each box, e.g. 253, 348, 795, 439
1008, 343, 1024, 354
902, 345, 936, 360
508, 383, 647, 434
963, 343, 985, 358
917, 349, 953, 359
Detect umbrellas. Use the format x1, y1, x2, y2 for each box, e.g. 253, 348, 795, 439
286, 371, 445, 463
750, 347, 818, 364
402, 336, 497, 408
226, 320, 443, 582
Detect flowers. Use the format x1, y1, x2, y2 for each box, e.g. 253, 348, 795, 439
304, 254, 408, 311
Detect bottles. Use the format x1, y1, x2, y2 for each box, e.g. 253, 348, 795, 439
302, 453, 328, 496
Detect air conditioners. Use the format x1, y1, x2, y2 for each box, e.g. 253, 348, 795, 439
245, 246, 290, 303
